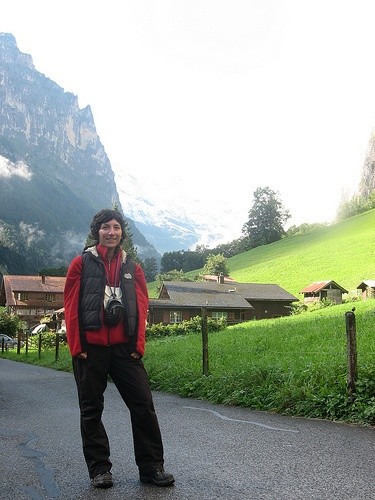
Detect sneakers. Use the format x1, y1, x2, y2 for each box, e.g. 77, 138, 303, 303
140, 469, 175, 485
91, 470, 113, 487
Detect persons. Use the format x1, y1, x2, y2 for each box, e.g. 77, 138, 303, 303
64, 209, 175, 486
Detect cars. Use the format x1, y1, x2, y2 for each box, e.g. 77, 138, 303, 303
0, 333, 25, 350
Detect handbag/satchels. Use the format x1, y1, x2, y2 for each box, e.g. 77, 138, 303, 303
103, 298, 126, 328
